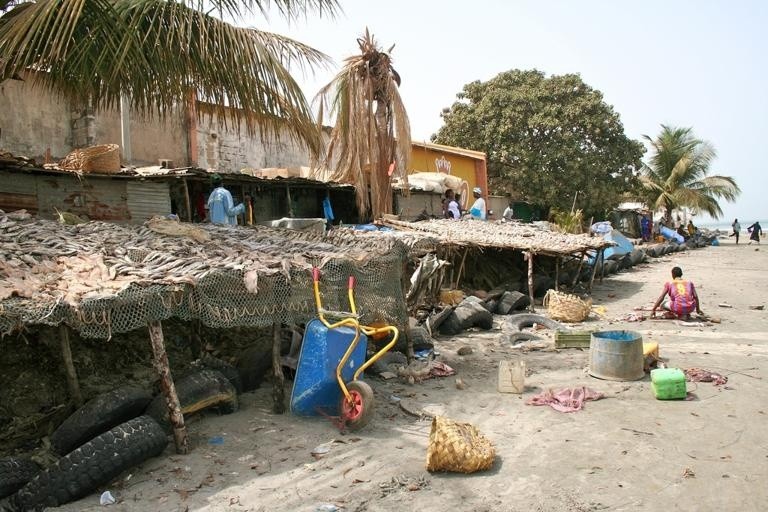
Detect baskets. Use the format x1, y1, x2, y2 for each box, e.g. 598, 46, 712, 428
75, 144, 121, 173
426, 415, 495, 474
542, 288, 591, 322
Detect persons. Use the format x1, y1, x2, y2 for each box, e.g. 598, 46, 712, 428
529, 211, 540, 222
453, 193, 463, 217
687, 220, 696, 236
502, 203, 514, 222
746, 221, 763, 245
441, 188, 463, 220
463, 188, 487, 222
731, 218, 742, 245
640, 215, 650, 242
206, 172, 252, 226
677, 225, 690, 242
648, 266, 705, 322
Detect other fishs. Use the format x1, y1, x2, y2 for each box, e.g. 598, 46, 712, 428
411, 216, 618, 253
0, 207, 397, 309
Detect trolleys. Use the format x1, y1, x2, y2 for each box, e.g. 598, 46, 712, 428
282, 264, 401, 432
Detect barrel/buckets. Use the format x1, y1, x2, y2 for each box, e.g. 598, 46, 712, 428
588, 330, 646, 379
648, 367, 687, 399
